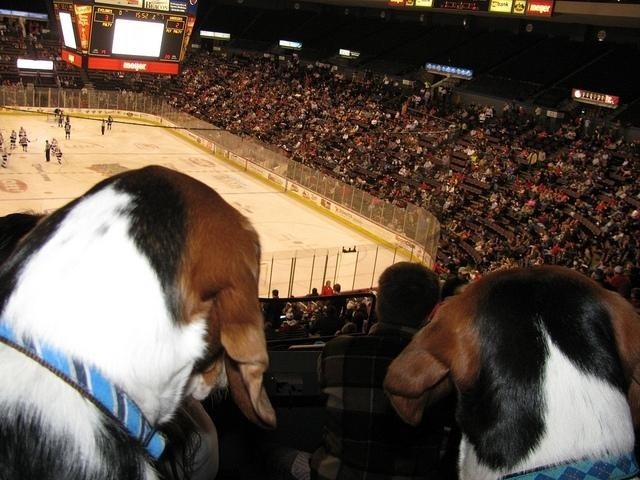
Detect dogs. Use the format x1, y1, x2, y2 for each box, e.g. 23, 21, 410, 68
384, 265, 640, 480
0, 165, 276, 480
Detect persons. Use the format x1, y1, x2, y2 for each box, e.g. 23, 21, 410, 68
0, 147, 12, 168
44, 140, 51, 162
50, 137, 58, 156
64, 120, 72, 140
19, 135, 31, 153
179, 258, 640, 480
0, 14, 155, 128
0, 131, 5, 152
9, 130, 17, 149
1, 213, 48, 267
55, 147, 63, 165
102, 118, 106, 135
18, 126, 27, 145
155, 34, 638, 257
107, 115, 113, 130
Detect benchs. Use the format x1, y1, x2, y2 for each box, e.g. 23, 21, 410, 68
253, 31, 640, 142
2, 30, 253, 142
236, 141, 640, 480
